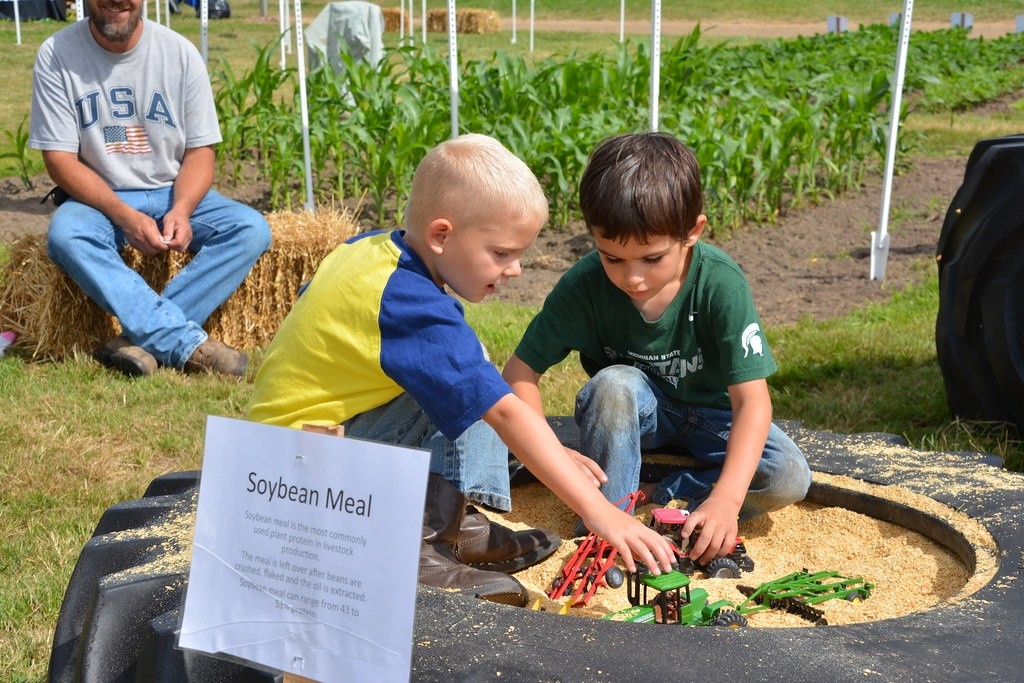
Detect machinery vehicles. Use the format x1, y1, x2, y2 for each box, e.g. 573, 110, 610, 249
594, 564, 874, 631
648, 507, 755, 580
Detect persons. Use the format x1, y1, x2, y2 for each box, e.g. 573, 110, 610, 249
28, 0, 269, 379
245, 134, 677, 608
502, 131, 811, 566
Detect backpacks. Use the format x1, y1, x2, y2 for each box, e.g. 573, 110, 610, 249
936, 133, 1024, 440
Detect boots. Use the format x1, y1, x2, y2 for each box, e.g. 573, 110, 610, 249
418, 473, 529, 608
456, 505, 563, 574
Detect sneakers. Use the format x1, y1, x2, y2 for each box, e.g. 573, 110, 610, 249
188, 339, 247, 376
93, 336, 157, 378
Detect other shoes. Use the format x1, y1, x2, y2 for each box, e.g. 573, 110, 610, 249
575, 518, 590, 537
